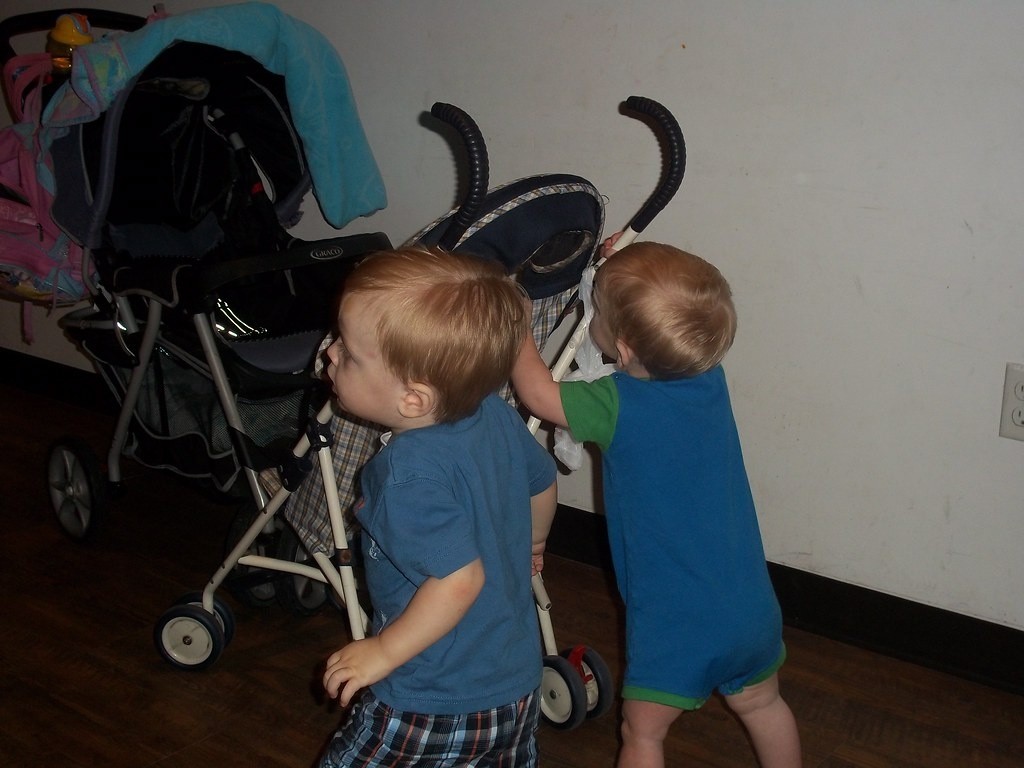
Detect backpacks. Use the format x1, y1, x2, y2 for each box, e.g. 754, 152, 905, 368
0, 52, 101, 344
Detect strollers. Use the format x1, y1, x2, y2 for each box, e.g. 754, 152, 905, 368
0, 7, 395, 609
149, 93, 688, 735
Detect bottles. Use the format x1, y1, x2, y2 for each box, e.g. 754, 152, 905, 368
46, 13, 91, 76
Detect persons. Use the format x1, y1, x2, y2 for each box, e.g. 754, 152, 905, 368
314, 245, 559, 768
509, 231, 802, 768
